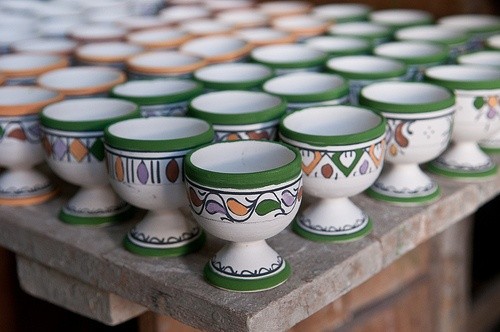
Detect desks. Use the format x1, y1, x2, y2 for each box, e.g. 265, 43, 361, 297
1, 0, 500, 331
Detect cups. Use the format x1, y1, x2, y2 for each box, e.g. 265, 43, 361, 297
0, 0, 500, 293
